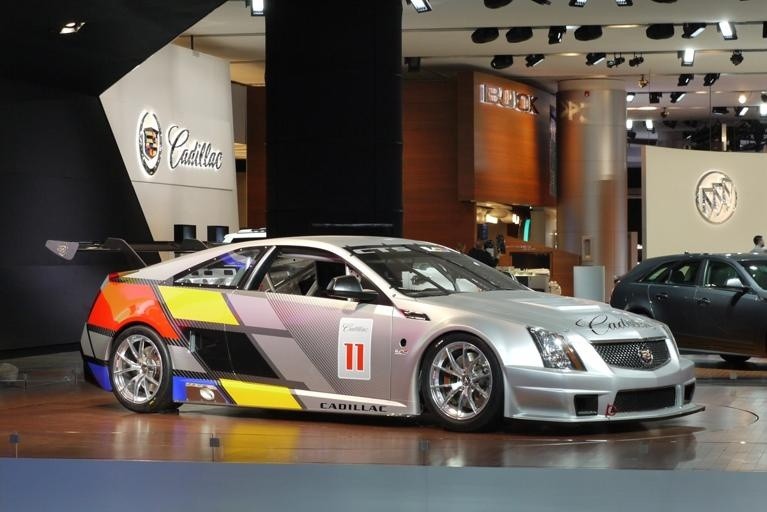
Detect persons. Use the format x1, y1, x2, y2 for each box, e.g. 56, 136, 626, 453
750, 235, 767, 253
468, 240, 507, 268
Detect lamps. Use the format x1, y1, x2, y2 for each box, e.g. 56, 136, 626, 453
245, 0, 264, 16
406, 0, 432, 13
475, 207, 520, 224
472, 0, 767, 134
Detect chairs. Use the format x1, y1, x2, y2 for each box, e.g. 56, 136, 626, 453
685, 268, 698, 282
663, 271, 685, 282
307, 260, 346, 297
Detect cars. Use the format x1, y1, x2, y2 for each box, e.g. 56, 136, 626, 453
43, 234, 704, 432
609, 253, 767, 365
